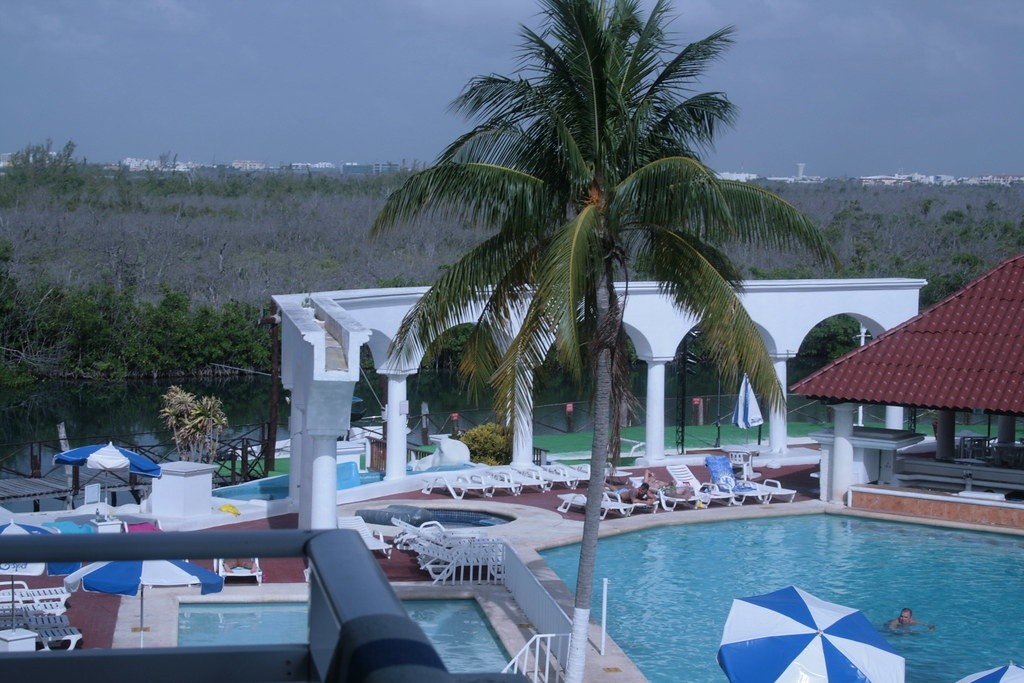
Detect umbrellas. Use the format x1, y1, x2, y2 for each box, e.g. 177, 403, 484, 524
52, 440, 163, 520
64, 560, 224, 648
0, 518, 82, 632
731, 373, 764, 444
715, 586, 906, 683
955, 660, 1024, 683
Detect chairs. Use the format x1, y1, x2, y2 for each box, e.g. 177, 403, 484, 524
39, 518, 166, 534
338, 516, 393, 560
213, 558, 262, 587
391, 517, 506, 580
421, 460, 632, 500
557, 455, 797, 520
0, 581, 83, 651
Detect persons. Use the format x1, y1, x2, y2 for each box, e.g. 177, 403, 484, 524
644, 469, 692, 499
603, 481, 656, 507
891, 607, 916, 624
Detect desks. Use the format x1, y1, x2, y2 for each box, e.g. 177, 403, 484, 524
0, 628, 38, 651
90, 517, 122, 533
